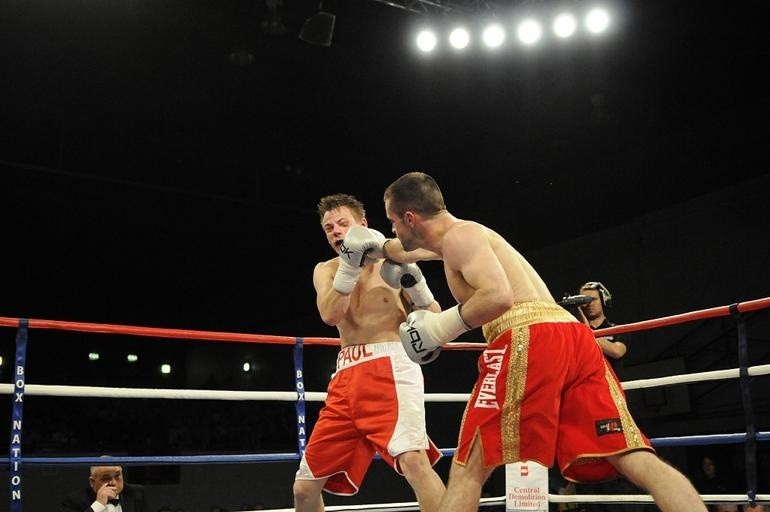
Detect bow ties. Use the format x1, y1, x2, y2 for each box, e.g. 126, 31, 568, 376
107, 498, 120, 507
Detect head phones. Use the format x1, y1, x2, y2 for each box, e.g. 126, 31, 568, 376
586, 280, 615, 307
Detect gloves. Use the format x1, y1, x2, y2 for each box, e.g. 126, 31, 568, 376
364, 228, 393, 265
399, 302, 473, 366
332, 225, 379, 294
380, 259, 435, 307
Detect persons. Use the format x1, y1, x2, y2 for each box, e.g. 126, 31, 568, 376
557, 477, 580, 512
567, 281, 631, 381
64, 456, 160, 512
692, 454, 723, 511
364, 172, 709, 512
293, 195, 447, 512
742, 504, 769, 512
717, 504, 739, 512
